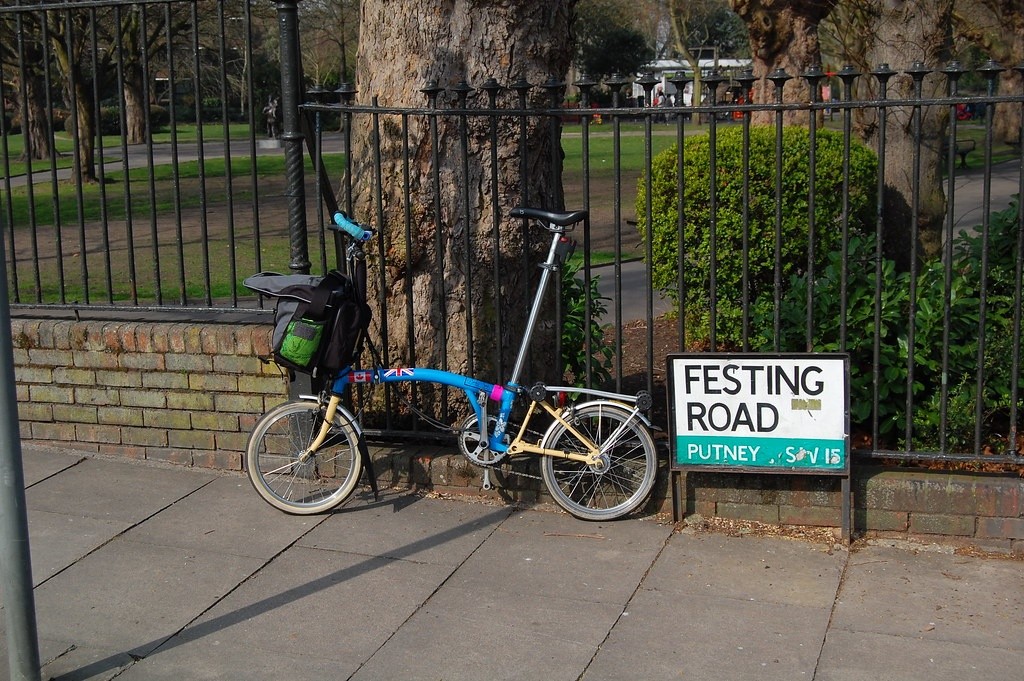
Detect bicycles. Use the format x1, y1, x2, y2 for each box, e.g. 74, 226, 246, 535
244, 205, 665, 522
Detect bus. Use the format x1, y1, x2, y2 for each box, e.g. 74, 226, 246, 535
634, 57, 753, 107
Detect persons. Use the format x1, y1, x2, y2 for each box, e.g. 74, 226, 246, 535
264, 93, 280, 140
652, 90, 672, 122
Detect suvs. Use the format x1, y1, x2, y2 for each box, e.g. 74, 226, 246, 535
155, 77, 204, 106
